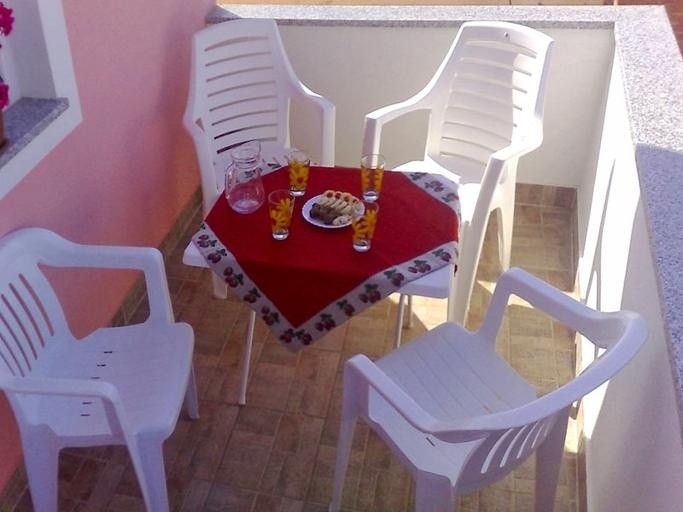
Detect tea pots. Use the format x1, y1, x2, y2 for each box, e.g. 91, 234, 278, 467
225, 141, 268, 215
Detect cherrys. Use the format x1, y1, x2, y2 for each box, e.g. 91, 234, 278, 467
410, 173, 459, 202
197, 235, 452, 346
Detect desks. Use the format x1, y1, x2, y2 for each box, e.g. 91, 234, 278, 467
183, 159, 460, 404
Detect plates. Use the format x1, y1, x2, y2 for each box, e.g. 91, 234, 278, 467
302, 192, 367, 229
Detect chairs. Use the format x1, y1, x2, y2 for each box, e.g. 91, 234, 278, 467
358, 17, 558, 352
325, 263, 647, 508
184, 17, 337, 299
0, 223, 204, 512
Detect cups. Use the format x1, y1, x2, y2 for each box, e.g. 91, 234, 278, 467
351, 200, 380, 252
288, 150, 310, 196
360, 154, 386, 202
269, 189, 296, 241
